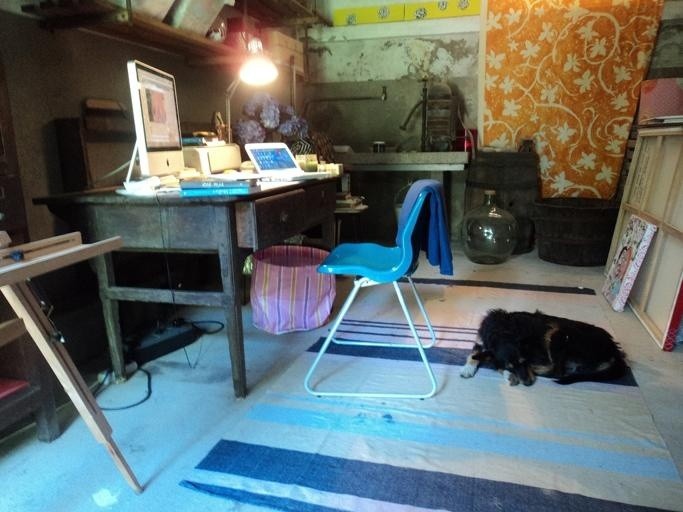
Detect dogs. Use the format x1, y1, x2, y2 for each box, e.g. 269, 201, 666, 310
460, 306, 627, 388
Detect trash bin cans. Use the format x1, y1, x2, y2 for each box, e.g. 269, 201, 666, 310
252, 246, 335, 333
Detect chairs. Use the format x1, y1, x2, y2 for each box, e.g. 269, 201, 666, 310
303, 192, 438, 401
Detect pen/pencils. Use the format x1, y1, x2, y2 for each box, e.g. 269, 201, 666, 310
260, 178, 294, 183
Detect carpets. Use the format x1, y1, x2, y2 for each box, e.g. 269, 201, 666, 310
153, 278, 683, 512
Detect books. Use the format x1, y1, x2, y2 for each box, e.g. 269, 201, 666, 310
178, 179, 264, 196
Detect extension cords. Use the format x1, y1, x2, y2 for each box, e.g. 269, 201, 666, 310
97, 360, 138, 383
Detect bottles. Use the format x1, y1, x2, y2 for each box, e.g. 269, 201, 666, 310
459, 189, 519, 266
372, 142, 385, 153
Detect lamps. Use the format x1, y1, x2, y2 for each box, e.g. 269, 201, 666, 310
226, 37, 279, 144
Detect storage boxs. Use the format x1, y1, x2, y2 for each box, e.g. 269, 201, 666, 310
115, 0, 235, 37
269, 31, 304, 68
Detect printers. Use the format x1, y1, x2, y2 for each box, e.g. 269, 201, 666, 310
183, 144, 242, 176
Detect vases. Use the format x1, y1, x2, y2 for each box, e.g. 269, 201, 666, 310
458, 191, 519, 265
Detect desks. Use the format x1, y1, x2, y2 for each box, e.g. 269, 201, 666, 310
336, 152, 470, 238
32, 179, 337, 398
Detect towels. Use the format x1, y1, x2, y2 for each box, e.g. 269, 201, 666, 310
396, 179, 454, 276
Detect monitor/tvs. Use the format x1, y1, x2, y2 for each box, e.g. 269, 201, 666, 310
126, 60, 185, 182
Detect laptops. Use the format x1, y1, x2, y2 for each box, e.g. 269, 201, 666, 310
245, 142, 331, 181
